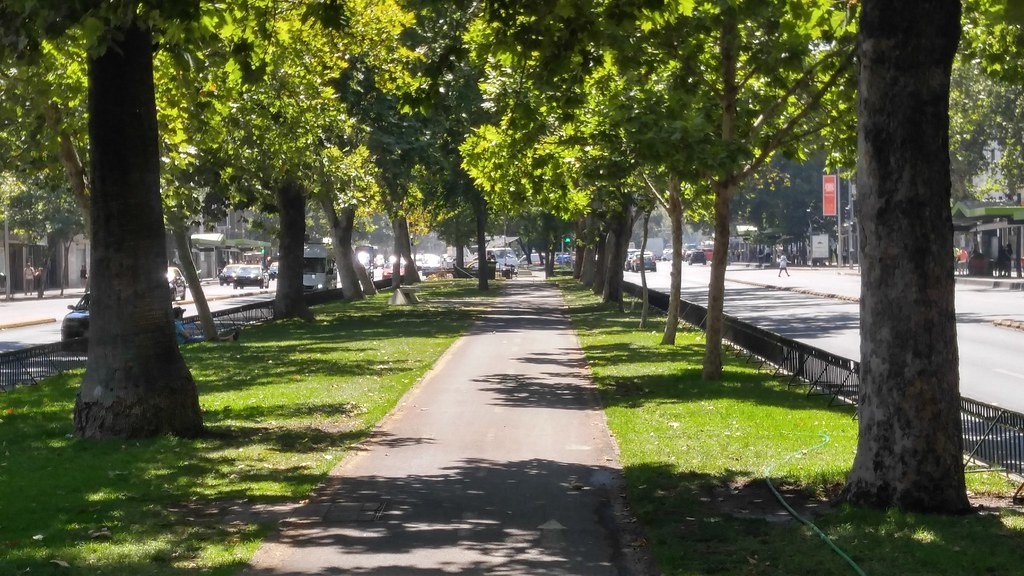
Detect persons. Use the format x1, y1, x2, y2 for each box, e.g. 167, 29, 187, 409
776, 252, 791, 277
735, 249, 740, 262
79, 264, 88, 292
173, 307, 239, 344
24, 263, 43, 295
828, 245, 848, 266
954, 242, 1013, 277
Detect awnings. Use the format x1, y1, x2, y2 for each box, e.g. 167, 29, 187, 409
190, 232, 272, 248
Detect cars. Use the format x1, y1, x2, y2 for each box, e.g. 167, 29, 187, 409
380, 261, 405, 279
60, 292, 90, 340
166, 266, 187, 303
267, 262, 279, 279
232, 265, 270, 289
662, 246, 714, 266
217, 264, 245, 286
519, 253, 550, 265
623, 248, 658, 274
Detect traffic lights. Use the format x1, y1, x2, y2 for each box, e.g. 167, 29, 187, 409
564, 236, 571, 243
844, 208, 850, 220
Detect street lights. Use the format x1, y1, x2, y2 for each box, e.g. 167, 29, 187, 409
832, 153, 842, 267
499, 217, 507, 266
806, 208, 814, 270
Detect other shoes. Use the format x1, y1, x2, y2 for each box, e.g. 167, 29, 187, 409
233, 330, 239, 340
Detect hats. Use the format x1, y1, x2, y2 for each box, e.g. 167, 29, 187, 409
174, 307, 186, 316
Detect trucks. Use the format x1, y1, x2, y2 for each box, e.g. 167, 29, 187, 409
422, 244, 520, 279
303, 241, 338, 294
637, 239, 664, 262
